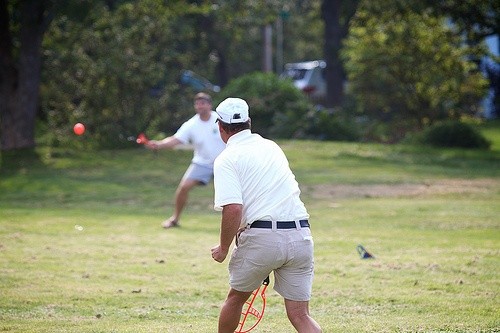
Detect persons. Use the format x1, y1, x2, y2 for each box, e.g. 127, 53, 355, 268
211, 97, 325, 333
144, 92, 231, 228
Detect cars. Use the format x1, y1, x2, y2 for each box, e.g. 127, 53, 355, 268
282, 60, 348, 103
148, 69, 222, 99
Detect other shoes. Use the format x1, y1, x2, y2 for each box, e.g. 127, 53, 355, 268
162, 218, 177, 228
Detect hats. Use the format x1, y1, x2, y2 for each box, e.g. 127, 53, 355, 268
194, 92, 212, 102
214, 97, 249, 124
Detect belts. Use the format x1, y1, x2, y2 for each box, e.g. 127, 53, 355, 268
249, 220, 311, 229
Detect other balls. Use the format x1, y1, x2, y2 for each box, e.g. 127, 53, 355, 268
74, 123, 85, 135
